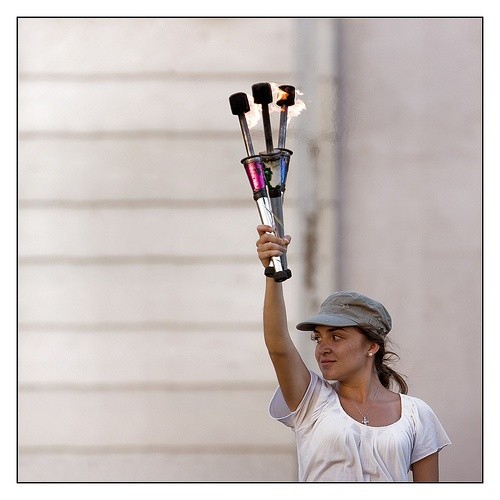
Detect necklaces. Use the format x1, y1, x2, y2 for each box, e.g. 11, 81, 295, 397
335, 383, 383, 427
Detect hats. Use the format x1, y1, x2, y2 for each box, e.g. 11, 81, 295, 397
295, 292, 393, 341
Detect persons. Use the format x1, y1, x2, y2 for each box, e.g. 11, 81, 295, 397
255, 221, 453, 485
